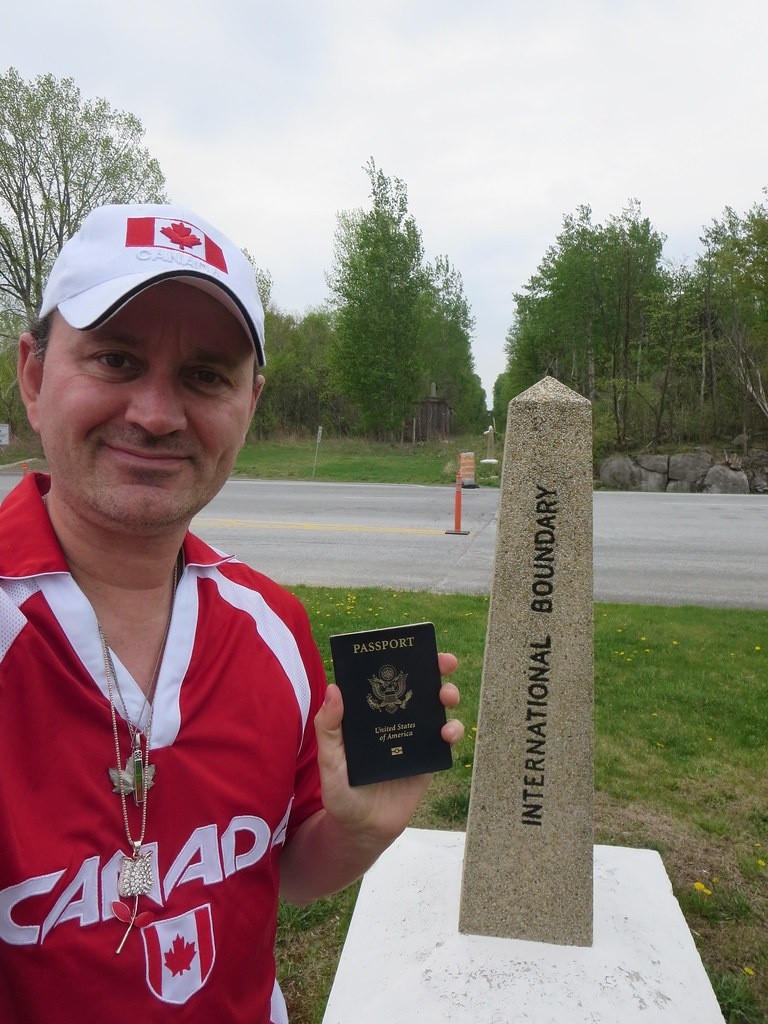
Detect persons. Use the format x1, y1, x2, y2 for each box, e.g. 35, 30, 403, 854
0, 202, 466, 1024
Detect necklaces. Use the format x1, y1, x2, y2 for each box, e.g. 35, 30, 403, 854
98, 555, 177, 954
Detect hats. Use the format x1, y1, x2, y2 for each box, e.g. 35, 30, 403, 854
38, 203, 266, 367
